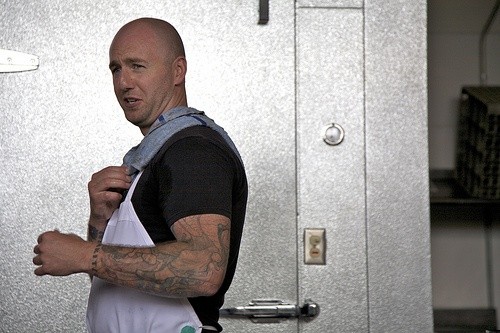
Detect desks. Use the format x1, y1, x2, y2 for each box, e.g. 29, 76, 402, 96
429, 169, 499, 222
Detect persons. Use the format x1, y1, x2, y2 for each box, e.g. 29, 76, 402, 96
32, 16, 247, 332
